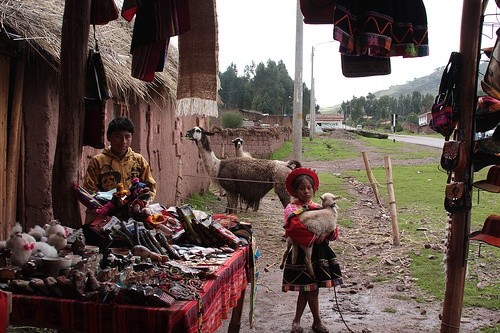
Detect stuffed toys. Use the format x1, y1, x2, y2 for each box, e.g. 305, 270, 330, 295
0, 220, 76, 267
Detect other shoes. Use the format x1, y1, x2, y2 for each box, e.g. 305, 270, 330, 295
292, 322, 302, 333
312, 322, 329, 333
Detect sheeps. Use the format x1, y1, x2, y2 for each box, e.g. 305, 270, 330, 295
231, 136, 253, 159
278, 192, 342, 279
185, 125, 303, 214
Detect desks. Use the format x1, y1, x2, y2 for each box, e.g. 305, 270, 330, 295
9, 236, 261, 333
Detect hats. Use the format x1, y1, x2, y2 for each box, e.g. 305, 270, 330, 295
472, 166, 500, 193
467, 215, 500, 248
472, 124, 500, 172
285, 168, 320, 198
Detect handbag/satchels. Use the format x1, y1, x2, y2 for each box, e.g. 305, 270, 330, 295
443, 182, 472, 213
429, 102, 457, 137
440, 141, 466, 172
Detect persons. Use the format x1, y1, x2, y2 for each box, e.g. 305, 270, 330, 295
83, 116, 156, 248
282, 167, 344, 333
